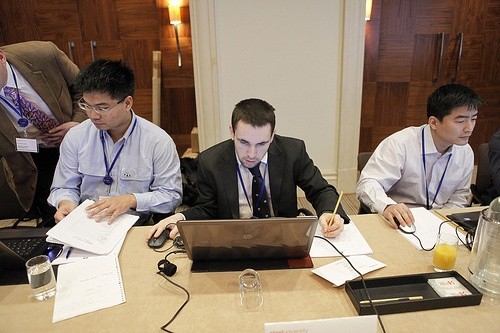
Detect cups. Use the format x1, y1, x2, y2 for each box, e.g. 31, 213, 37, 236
472, 208, 500, 299
433, 233, 460, 272
468, 207, 489, 277
26, 254, 58, 301
238, 268, 263, 312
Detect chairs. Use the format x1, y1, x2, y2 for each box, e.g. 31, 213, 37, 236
0, 146, 198, 229
357, 143, 494, 215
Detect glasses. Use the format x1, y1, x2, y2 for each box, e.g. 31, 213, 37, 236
77, 96, 126, 114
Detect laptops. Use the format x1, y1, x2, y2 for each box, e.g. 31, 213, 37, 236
450, 211, 481, 231
177, 216, 318, 261
0, 227, 52, 273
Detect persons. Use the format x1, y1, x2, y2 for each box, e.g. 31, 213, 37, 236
47, 59, 183, 223
0, 40, 89, 225
355, 84, 479, 228
487, 130, 500, 198
146, 99, 350, 244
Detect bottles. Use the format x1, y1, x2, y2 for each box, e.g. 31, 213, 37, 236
488, 196, 500, 218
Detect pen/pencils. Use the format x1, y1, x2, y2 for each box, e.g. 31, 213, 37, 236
434, 208, 464, 231
324, 191, 344, 237
66, 247, 73, 259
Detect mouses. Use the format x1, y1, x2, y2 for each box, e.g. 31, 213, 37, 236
394, 217, 416, 234
147, 228, 171, 248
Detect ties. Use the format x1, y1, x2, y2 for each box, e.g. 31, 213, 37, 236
248, 161, 271, 219
4, 86, 61, 148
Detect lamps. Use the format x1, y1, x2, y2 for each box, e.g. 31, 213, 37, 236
156, 0, 189, 67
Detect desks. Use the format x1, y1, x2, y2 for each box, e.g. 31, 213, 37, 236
0, 206, 500, 333
